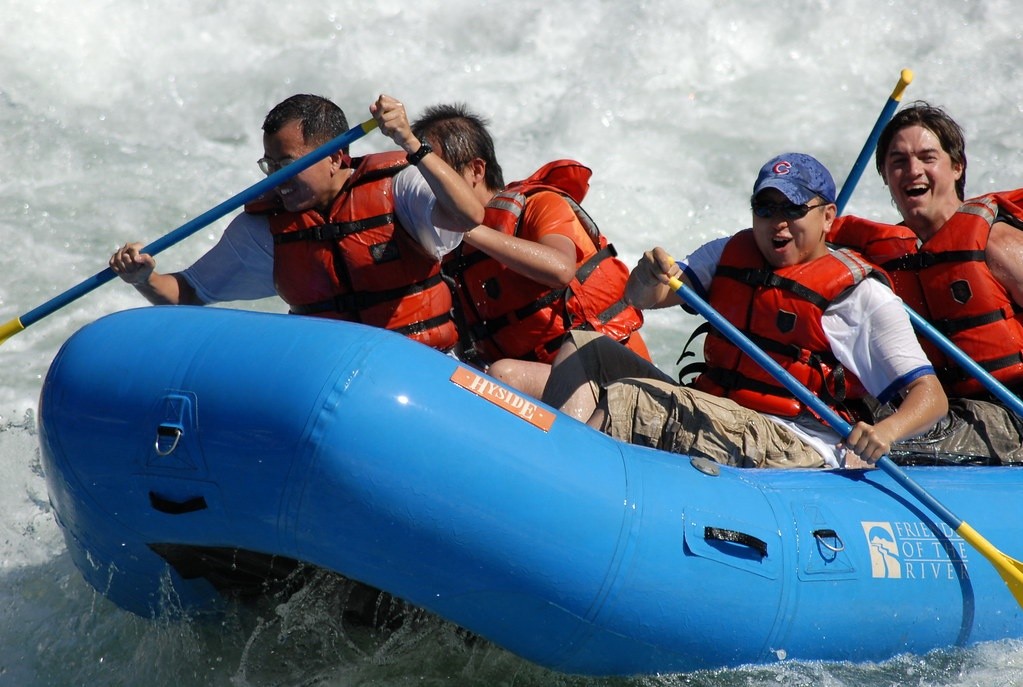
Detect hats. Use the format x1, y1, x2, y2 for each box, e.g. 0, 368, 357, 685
753, 152, 836, 205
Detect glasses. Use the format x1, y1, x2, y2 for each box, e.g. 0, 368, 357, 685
750, 196, 827, 219
258, 158, 296, 176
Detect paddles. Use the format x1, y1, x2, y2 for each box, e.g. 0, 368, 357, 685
1, 104, 383, 348
895, 289, 1023, 418
837, 71, 913, 213
669, 271, 1023, 616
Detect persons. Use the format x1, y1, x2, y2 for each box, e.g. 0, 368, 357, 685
538, 153, 949, 470
108, 92, 485, 362
409, 100, 653, 400
825, 100, 1022, 465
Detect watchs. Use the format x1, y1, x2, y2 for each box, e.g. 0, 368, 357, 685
405, 136, 434, 166
